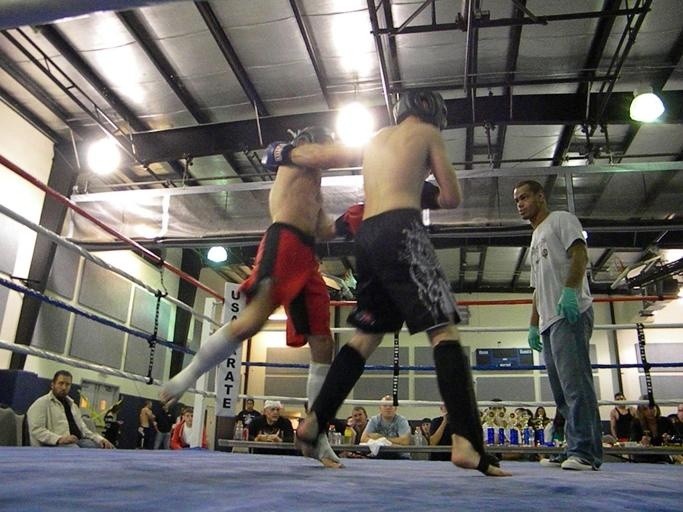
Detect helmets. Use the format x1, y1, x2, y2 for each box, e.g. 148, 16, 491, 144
292, 126, 338, 144
393, 89, 448, 129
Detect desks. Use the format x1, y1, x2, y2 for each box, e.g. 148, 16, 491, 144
217, 438, 682, 455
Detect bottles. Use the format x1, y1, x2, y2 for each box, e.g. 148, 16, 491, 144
233, 421, 248, 440
414, 426, 422, 445
486, 426, 544, 446
344, 426, 353, 445
544, 441, 556, 446
327, 424, 335, 444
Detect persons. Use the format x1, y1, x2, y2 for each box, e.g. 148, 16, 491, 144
103, 399, 204, 451
512, 179, 603, 470
262, 87, 512, 476
26, 370, 116, 449
161, 126, 356, 468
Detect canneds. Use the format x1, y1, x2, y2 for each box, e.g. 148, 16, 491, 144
242, 428, 248, 441
328, 431, 341, 445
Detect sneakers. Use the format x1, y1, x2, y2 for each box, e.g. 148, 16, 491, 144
540, 455, 568, 467
561, 456, 593, 470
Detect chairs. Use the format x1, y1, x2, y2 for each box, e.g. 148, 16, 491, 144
0, 401, 30, 446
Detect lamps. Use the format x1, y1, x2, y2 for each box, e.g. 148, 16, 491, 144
619, 0, 666, 124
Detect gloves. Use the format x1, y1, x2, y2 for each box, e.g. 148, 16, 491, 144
335, 204, 364, 241
422, 181, 440, 210
556, 286, 581, 325
261, 141, 293, 170
527, 324, 542, 353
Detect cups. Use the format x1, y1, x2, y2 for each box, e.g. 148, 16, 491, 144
333, 433, 340, 444
